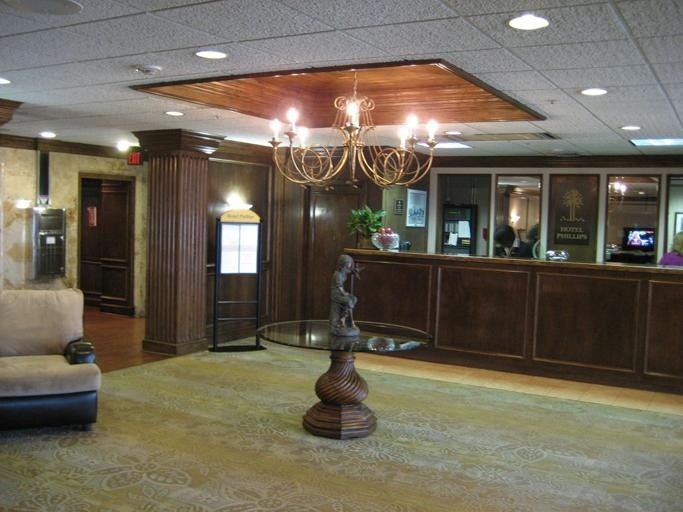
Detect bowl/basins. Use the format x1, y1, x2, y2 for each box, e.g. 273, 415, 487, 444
372, 234, 398, 252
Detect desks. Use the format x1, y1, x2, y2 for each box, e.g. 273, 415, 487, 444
256, 317, 436, 436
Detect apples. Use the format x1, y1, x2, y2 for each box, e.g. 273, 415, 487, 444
378, 227, 394, 233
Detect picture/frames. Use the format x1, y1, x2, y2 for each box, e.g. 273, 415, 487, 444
403, 187, 429, 231
546, 171, 600, 263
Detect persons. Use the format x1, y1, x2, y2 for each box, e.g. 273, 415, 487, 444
658, 232, 683, 267
330, 255, 361, 330
627, 231, 642, 245
494, 224, 533, 257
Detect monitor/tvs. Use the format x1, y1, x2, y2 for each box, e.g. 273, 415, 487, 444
623, 228, 656, 249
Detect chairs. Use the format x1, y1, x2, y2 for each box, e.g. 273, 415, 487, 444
1, 285, 103, 431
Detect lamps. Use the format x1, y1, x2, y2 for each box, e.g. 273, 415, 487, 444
269, 67, 440, 194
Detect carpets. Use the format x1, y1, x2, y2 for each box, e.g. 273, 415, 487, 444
2, 334, 682, 511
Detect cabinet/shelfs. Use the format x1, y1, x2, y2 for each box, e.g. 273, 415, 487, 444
439, 202, 479, 255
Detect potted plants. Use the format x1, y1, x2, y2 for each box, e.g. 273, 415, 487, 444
344, 200, 388, 247
